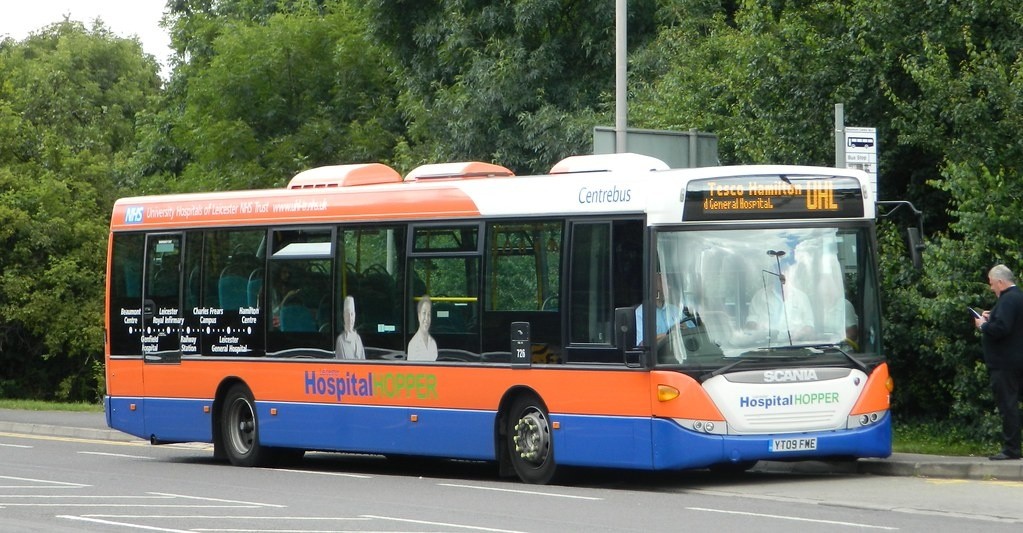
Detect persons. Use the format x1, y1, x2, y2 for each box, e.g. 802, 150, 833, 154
745, 259, 814, 341
974, 264, 1023, 460
270, 262, 302, 315
334, 296, 365, 360
820, 277, 859, 343
407, 295, 438, 362
634, 277, 696, 346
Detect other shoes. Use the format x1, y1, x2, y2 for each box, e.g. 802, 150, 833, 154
989, 452, 1012, 460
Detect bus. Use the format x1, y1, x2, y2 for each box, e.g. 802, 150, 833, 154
102, 151, 927, 485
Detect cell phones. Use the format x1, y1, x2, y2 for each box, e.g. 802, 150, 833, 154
969, 308, 983, 319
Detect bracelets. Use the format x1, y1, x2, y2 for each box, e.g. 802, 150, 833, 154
979, 325, 982, 332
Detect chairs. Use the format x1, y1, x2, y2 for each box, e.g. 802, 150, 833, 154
542, 294, 560, 312
154, 243, 469, 333
704, 295, 725, 314
